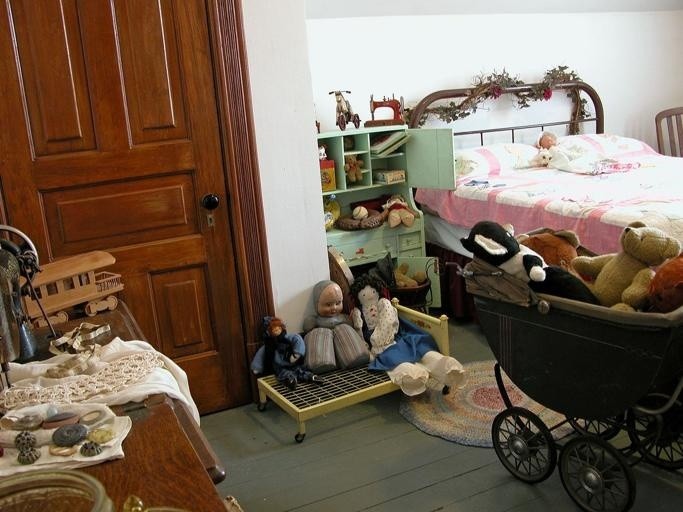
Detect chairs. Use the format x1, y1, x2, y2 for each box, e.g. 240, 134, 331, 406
654, 105, 683, 157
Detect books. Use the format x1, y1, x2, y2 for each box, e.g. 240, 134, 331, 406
371, 135, 410, 160
370, 132, 405, 154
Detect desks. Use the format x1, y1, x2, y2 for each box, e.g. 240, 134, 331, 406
0, 292, 230, 511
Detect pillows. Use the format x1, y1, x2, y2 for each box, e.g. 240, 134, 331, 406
454, 142, 550, 182
548, 134, 662, 176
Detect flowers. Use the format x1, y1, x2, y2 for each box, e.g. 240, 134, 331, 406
403, 63, 592, 136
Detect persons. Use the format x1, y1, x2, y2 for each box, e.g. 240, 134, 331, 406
304, 280, 369, 373
383, 194, 420, 227
251, 317, 324, 388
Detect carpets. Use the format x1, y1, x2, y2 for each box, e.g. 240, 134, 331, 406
399, 356, 587, 447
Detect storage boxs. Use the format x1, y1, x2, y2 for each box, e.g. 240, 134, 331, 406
318, 160, 337, 193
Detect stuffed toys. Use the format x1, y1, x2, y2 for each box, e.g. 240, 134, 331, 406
246, 192, 466, 397
465, 219, 683, 314
350, 278, 466, 395
343, 154, 363, 182
537, 132, 575, 164
394, 263, 427, 287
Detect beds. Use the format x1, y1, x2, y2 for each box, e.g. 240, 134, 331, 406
404, 80, 683, 321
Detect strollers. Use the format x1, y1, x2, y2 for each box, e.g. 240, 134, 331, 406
449, 228, 682, 512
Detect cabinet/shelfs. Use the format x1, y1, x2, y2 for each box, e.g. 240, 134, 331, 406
316, 124, 455, 318
